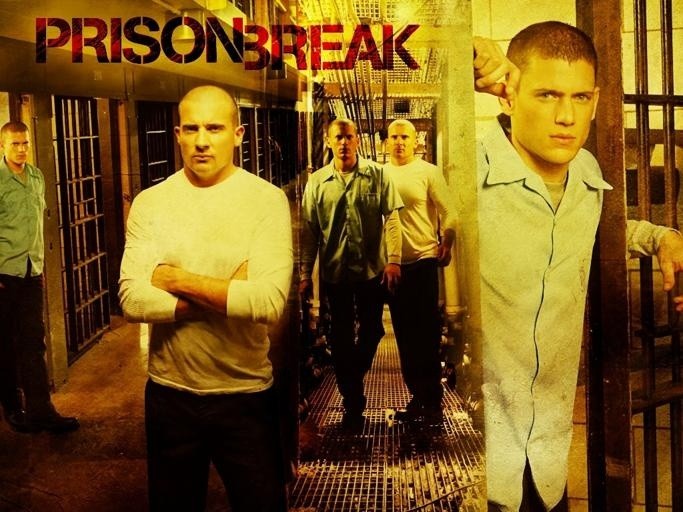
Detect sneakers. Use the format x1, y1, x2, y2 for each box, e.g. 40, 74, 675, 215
5, 408, 80, 434
341, 401, 446, 423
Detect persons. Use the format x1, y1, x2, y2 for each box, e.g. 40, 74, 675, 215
374, 120, 458, 428
1, 123, 81, 433
116, 84, 296, 511
470, 19, 682, 510
297, 117, 402, 435
